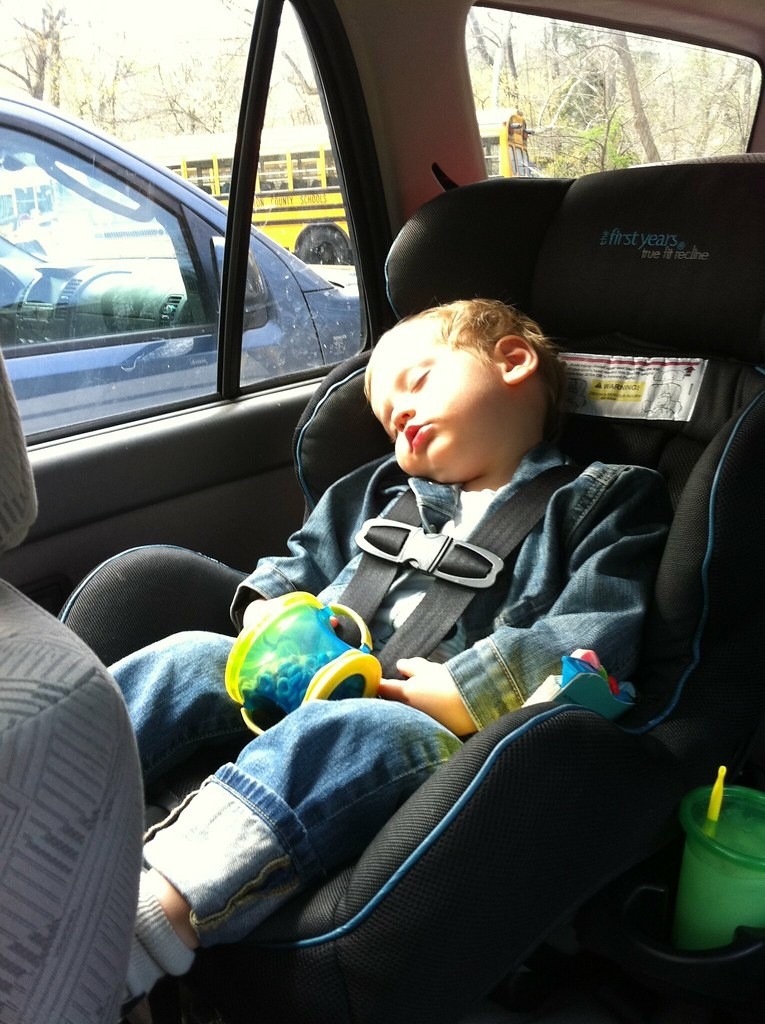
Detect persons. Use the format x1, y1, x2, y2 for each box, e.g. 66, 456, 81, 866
109, 296, 676, 1024
197, 180, 212, 194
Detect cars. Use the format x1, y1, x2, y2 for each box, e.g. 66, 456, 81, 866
1, 86, 362, 449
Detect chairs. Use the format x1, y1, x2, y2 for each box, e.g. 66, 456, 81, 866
51, 150, 765, 1023
0, 344, 145, 1024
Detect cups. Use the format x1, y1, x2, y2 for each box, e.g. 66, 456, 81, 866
669, 783, 765, 950
224, 590, 383, 738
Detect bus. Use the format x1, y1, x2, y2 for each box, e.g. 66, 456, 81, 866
53, 107, 532, 267
